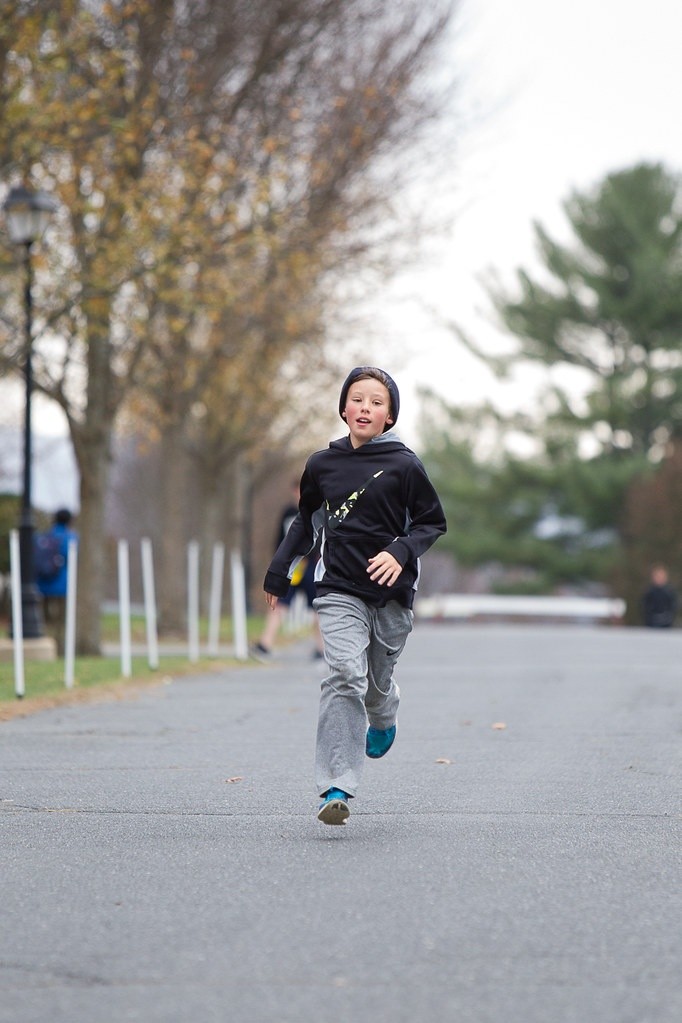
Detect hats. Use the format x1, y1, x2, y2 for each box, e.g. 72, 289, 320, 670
339, 367, 400, 433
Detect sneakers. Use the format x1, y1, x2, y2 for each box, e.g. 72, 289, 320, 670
317, 787, 351, 826
366, 721, 397, 759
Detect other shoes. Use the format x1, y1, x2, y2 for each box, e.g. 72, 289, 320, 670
310, 649, 325, 663
247, 643, 278, 667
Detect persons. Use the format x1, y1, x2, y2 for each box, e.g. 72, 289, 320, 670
34, 507, 77, 658
642, 564, 680, 629
262, 366, 447, 825
248, 478, 325, 662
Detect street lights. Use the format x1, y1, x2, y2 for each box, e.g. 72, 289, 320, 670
2, 176, 60, 640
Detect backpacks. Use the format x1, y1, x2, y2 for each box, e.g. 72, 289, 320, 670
33, 532, 64, 583
